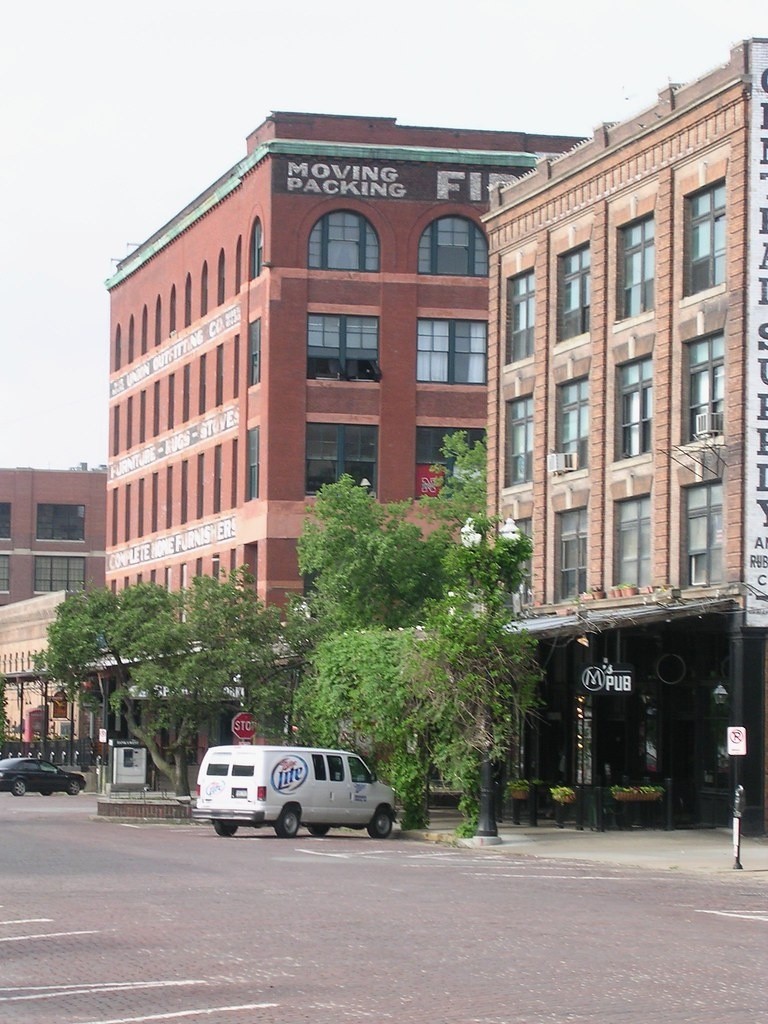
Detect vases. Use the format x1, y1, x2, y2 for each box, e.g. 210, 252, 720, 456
617, 791, 661, 800
561, 794, 574, 804
511, 787, 527, 799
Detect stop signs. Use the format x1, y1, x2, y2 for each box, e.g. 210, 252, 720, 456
232, 712, 259, 740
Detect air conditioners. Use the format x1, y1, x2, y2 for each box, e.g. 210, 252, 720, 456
696, 411, 724, 435
547, 449, 577, 474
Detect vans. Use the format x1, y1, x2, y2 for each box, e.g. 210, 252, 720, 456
190, 742, 398, 840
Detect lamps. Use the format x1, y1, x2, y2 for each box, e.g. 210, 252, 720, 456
713, 679, 729, 704
578, 633, 589, 649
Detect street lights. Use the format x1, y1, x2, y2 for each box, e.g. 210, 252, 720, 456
714, 682, 743, 869
460, 514, 522, 847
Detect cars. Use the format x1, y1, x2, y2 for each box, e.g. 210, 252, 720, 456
0, 758, 86, 797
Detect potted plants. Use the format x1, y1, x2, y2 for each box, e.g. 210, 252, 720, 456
579, 582, 640, 600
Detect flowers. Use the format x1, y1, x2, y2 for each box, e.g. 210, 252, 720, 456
551, 786, 575, 805
611, 784, 663, 796
505, 777, 542, 791
